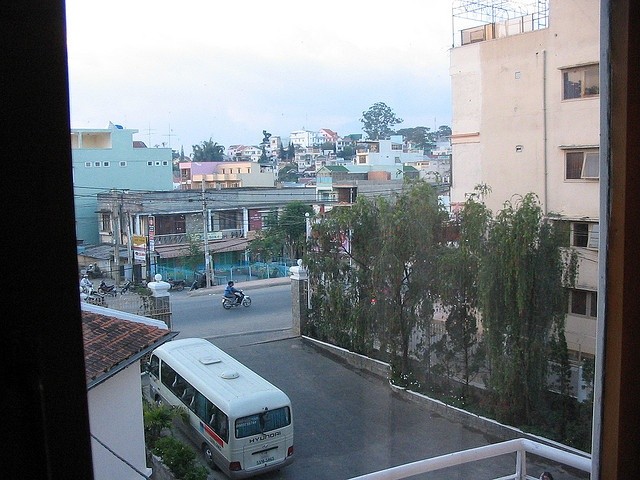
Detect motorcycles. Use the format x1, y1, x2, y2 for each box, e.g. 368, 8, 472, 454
120, 277, 149, 294
166, 278, 185, 293
98, 279, 118, 297
83, 270, 102, 280
80, 283, 104, 304
221, 289, 251, 310
190, 272, 212, 291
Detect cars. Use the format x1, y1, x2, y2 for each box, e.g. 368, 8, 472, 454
243, 262, 278, 278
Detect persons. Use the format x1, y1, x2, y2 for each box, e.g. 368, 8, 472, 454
81, 274, 92, 293
224, 281, 236, 304
231, 280, 243, 304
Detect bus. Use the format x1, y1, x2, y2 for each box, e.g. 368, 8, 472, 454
146, 337, 294, 479
